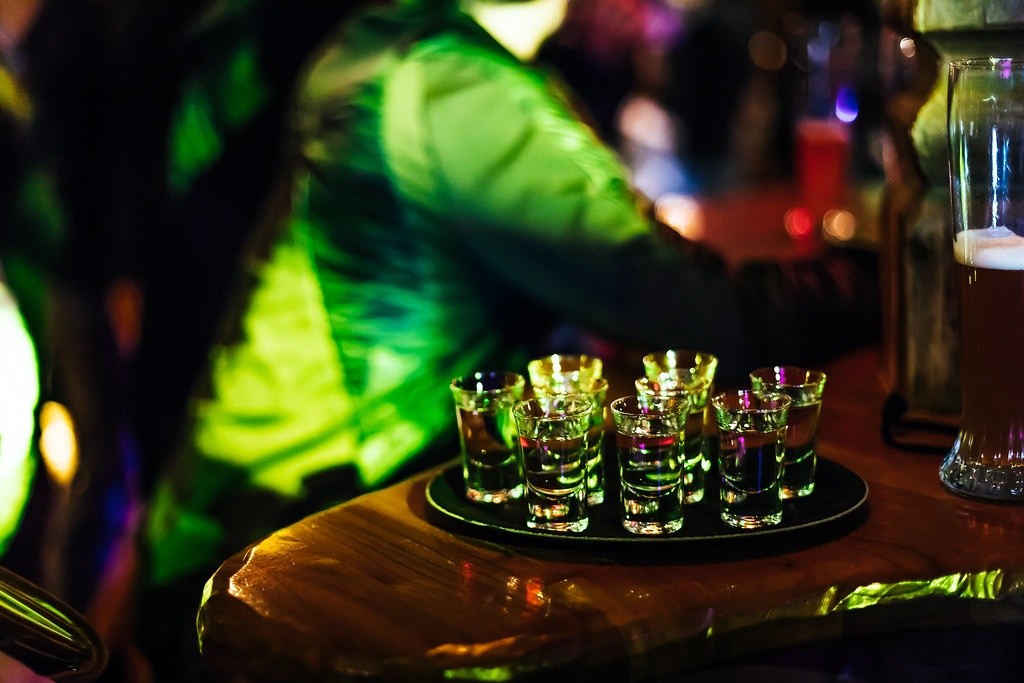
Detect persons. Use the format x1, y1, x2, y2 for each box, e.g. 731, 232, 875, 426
125, 0, 885, 683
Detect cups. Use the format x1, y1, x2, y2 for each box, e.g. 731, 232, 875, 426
611, 351, 718, 534
451, 372, 526, 503
938, 55, 1024, 501
712, 390, 791, 529
752, 366, 828, 500
513, 354, 608, 534
